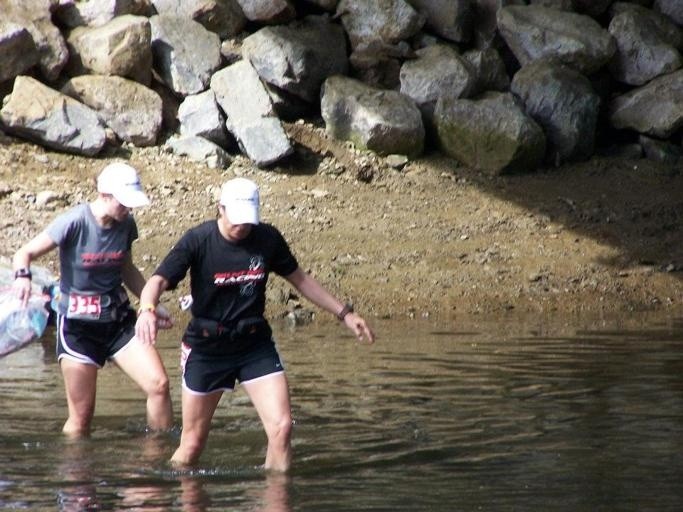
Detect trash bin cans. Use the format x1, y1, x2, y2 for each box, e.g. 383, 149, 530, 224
13, 267, 31, 280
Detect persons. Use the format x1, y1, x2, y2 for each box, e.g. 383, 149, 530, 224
133, 178, 374, 465
11, 162, 174, 433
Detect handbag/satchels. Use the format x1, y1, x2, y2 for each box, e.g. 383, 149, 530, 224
337, 303, 353, 321
137, 304, 158, 314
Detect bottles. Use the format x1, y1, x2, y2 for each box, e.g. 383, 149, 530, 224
97, 162, 151, 209
219, 177, 262, 226
